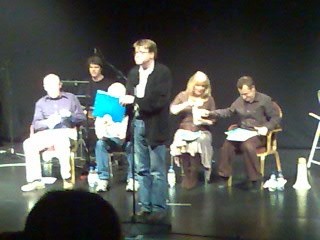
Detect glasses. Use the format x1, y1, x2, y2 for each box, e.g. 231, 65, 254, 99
136, 51, 150, 55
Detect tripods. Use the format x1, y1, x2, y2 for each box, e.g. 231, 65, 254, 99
120, 119, 171, 229
77, 126, 89, 169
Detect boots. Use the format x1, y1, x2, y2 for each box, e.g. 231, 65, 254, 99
189, 154, 199, 189
180, 152, 191, 187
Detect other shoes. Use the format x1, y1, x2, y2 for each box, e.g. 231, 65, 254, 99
249, 181, 261, 193
21, 181, 45, 192
148, 212, 165, 222
63, 179, 74, 190
126, 179, 139, 192
135, 211, 149, 219
217, 175, 227, 189
96, 180, 109, 192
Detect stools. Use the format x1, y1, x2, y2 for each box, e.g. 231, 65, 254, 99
108, 151, 127, 178
171, 149, 209, 184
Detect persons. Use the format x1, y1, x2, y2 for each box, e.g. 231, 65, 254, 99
198, 76, 281, 188
79, 56, 112, 178
120, 39, 175, 215
23, 187, 124, 240
89, 82, 129, 192
20, 74, 87, 190
169, 70, 216, 181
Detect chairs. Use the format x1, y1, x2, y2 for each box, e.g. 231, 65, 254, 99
29, 124, 79, 184
227, 124, 283, 186
